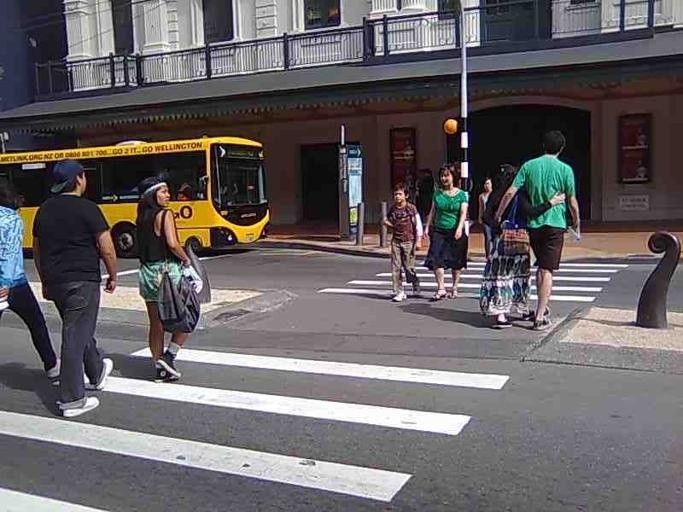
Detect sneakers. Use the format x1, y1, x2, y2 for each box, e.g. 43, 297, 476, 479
413, 283, 420, 294
154, 365, 177, 382
522, 309, 535, 321
535, 306, 551, 316
394, 291, 406, 303
533, 316, 552, 329
494, 319, 514, 329
47, 357, 63, 381
63, 397, 99, 418
157, 350, 182, 376
96, 359, 114, 390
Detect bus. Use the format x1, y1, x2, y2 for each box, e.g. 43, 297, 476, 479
0, 131, 273, 258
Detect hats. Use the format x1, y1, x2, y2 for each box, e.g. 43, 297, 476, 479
48, 159, 85, 193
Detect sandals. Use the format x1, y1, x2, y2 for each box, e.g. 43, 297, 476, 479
450, 288, 457, 299
431, 288, 447, 301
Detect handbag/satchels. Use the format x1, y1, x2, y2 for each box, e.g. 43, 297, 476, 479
156, 272, 200, 334
500, 229, 530, 256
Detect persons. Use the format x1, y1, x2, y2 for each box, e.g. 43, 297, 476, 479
0, 177, 60, 389
383, 182, 423, 302
135, 176, 190, 383
31, 158, 119, 418
476, 177, 500, 260
176, 181, 194, 201
478, 164, 566, 327
414, 167, 474, 223
422, 163, 468, 302
494, 127, 580, 331
